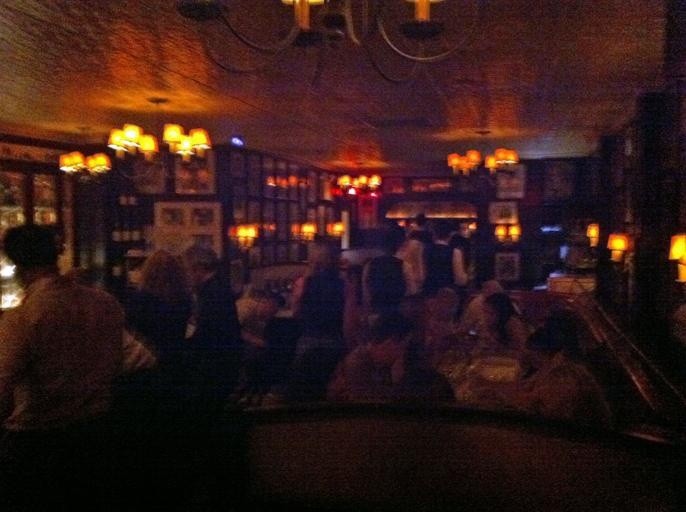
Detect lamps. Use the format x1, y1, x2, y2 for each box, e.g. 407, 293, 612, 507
106, 0, 485, 183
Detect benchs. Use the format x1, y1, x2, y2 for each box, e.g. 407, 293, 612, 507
231, 291, 686, 512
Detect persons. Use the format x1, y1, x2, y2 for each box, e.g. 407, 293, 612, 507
0, 208, 635, 512
